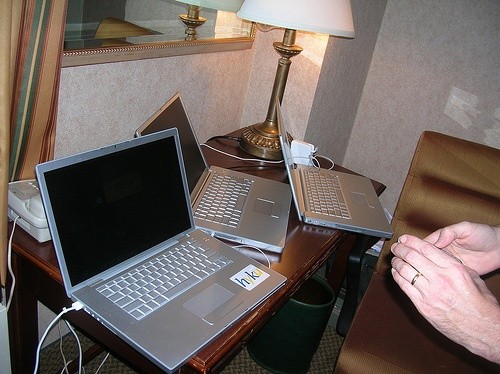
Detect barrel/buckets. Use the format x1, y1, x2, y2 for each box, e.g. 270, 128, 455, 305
248, 274, 336, 374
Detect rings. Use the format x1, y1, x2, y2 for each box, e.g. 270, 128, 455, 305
411, 273, 421, 285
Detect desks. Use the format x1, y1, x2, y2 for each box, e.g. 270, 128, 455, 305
7, 127, 388, 374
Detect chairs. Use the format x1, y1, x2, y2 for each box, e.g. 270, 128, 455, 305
338, 131, 500, 374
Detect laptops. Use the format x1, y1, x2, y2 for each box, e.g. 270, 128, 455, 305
275, 95, 393, 239
34, 128, 288, 373
134, 91, 292, 253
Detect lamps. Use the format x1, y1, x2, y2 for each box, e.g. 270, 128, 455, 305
234, 0, 357, 161
174, 0, 243, 43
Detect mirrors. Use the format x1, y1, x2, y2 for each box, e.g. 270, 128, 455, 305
61, 0, 258, 70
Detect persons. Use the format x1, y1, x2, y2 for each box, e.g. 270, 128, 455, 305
390, 221, 500, 364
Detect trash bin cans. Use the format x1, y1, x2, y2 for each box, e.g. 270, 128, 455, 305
246, 275, 336, 374
